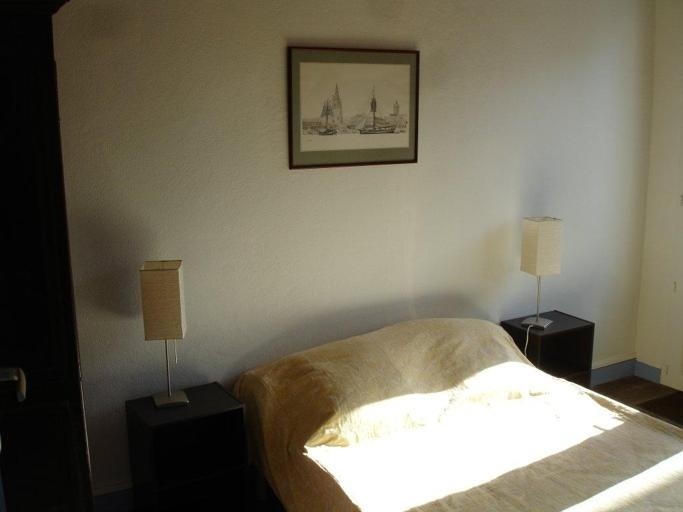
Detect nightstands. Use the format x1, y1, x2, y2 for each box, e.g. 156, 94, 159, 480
126, 383, 245, 512
502, 311, 594, 389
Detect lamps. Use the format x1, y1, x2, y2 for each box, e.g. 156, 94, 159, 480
520, 217, 562, 329
139, 260, 189, 406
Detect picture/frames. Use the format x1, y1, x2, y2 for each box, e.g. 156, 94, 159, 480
287, 45, 419, 170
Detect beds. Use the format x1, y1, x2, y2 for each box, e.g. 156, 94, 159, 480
233, 318, 683, 512
